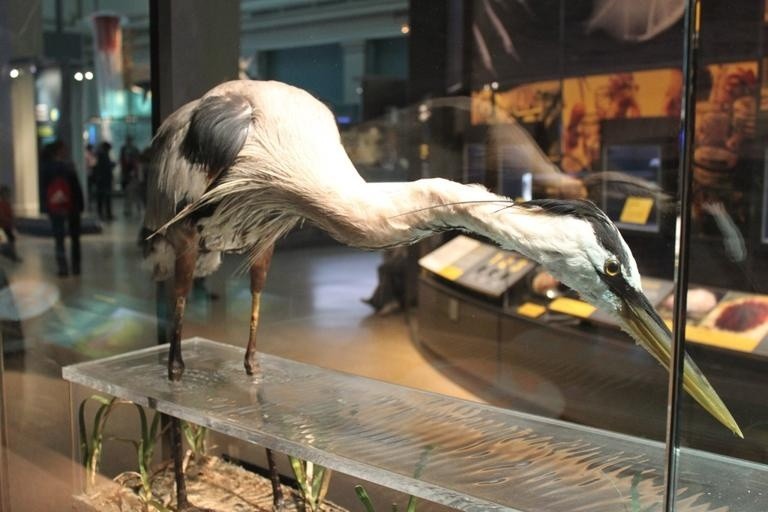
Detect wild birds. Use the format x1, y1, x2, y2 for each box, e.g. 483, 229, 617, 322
133, 77, 745, 512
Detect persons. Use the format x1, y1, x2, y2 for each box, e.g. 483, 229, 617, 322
87, 131, 143, 222
0, 185, 15, 242
41, 138, 85, 278
